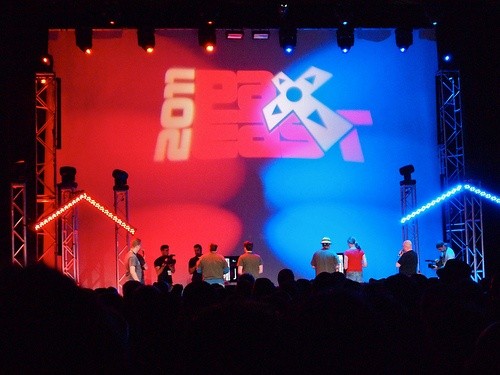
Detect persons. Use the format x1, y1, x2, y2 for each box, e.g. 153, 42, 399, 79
0, 238, 500, 375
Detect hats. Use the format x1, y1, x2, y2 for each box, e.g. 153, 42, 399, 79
321, 237, 331, 244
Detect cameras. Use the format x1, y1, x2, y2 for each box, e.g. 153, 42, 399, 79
164, 255, 176, 264
426, 258, 442, 268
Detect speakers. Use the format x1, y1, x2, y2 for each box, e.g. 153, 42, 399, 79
400, 165, 414, 175
112, 170, 129, 185
60, 167, 76, 182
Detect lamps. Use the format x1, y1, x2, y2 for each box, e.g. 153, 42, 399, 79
55, 166, 78, 187
75, 23, 92, 56
226, 29, 244, 41
197, 15, 218, 54
251, 29, 270, 41
400, 164, 417, 185
396, 22, 414, 52
336, 8, 356, 48
279, 12, 299, 56
112, 169, 129, 191
137, 23, 156, 55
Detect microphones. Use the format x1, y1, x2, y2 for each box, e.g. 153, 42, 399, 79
397, 249, 403, 257
